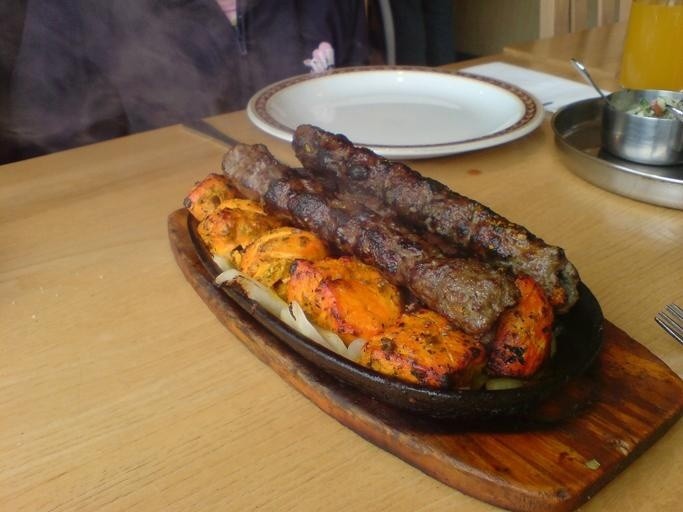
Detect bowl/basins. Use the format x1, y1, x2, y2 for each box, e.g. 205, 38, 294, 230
601, 87, 681, 166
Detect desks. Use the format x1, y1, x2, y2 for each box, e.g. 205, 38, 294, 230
503, 19, 626, 79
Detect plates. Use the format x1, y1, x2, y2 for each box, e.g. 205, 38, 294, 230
248, 59, 542, 163
189, 199, 605, 415
548, 97, 683, 215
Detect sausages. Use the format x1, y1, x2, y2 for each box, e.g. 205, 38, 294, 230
221, 143, 520, 343
290, 125, 580, 315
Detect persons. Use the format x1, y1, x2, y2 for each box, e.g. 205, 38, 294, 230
0, 0, 428, 155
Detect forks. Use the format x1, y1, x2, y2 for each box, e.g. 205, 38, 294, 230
651, 297, 683, 345
